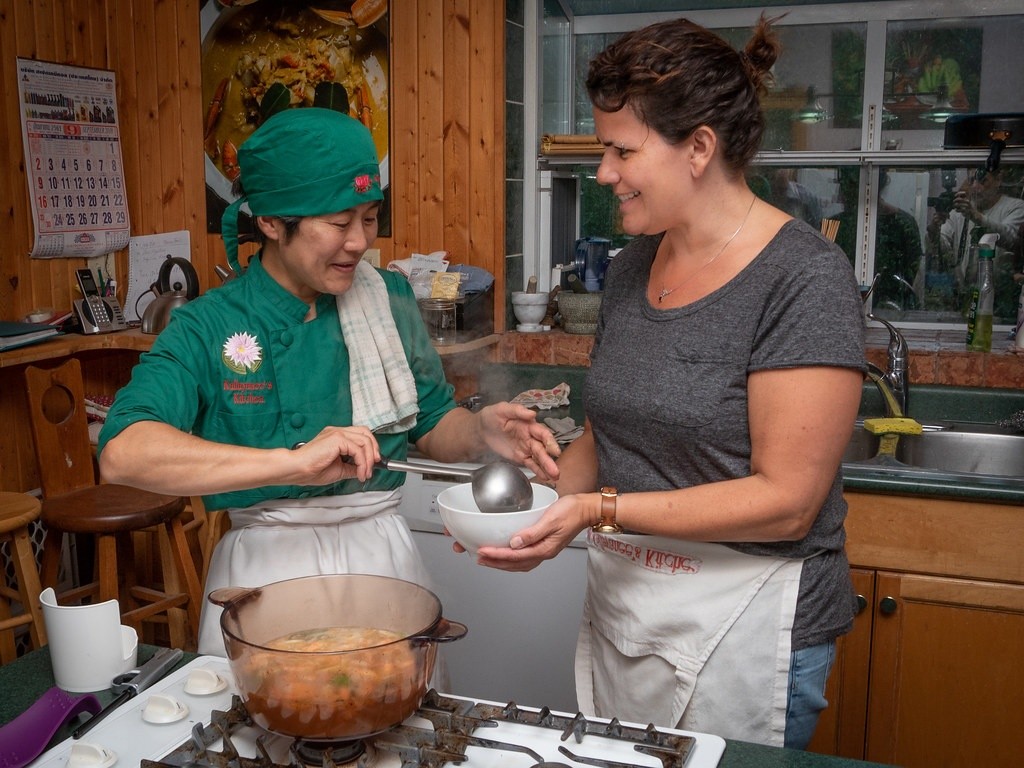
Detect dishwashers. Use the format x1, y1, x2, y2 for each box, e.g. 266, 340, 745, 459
399, 458, 589, 716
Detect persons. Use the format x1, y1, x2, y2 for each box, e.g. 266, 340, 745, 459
926, 169, 1024, 317
97, 108, 559, 694
827, 148, 923, 308
445, 18, 870, 752
764, 168, 823, 229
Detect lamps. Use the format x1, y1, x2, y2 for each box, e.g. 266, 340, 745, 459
790, 69, 958, 123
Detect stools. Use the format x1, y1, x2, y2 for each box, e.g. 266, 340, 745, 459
0, 483, 215, 667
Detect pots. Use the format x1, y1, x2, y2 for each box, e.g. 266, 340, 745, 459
939, 112, 1024, 173
207, 574, 470, 742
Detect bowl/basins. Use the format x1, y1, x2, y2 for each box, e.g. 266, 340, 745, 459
201, 1, 390, 219
437, 481, 560, 560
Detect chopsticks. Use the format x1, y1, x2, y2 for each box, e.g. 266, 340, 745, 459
820, 219, 841, 244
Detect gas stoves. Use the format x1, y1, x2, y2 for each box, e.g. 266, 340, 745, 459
28, 655, 726, 767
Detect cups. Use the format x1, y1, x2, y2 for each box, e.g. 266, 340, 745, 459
421, 298, 457, 346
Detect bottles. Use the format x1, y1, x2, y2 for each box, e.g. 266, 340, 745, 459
965, 247, 995, 352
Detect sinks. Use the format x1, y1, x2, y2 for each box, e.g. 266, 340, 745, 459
841, 427, 880, 462
896, 430, 1024, 476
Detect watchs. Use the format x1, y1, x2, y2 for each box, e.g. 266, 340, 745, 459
593, 488, 623, 535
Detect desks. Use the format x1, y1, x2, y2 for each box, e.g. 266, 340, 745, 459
0, 307, 499, 369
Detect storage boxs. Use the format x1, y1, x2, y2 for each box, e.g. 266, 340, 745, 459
432, 281, 494, 329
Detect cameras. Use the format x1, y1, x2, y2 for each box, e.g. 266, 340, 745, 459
927, 165, 959, 212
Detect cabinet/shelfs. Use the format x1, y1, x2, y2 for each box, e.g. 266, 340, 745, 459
807, 492, 1023, 768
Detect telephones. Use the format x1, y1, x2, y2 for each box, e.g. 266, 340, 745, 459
72, 269, 129, 335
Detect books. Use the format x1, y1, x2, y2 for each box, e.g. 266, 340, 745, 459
122, 229, 191, 321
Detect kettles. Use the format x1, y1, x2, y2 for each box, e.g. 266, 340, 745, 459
141, 257, 200, 334
214, 256, 235, 285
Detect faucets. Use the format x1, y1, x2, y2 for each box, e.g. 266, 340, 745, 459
861, 313, 954, 431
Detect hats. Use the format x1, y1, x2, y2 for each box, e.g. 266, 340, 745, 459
222, 108, 386, 276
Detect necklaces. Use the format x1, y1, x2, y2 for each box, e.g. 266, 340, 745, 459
659, 193, 756, 302
